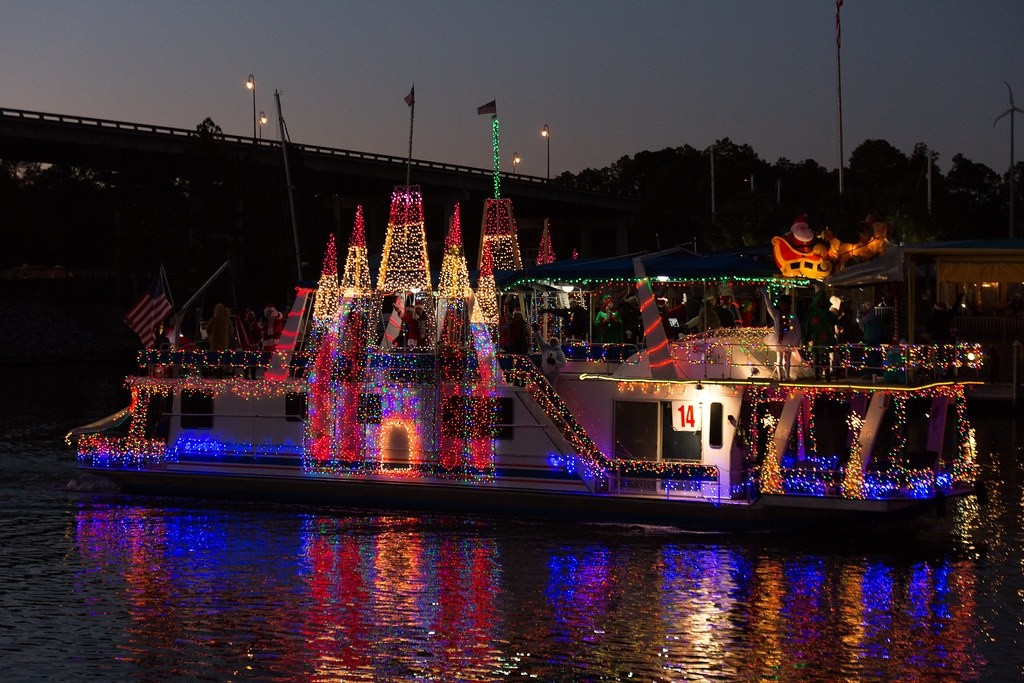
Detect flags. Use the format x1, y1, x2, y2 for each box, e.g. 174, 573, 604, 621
832, 0, 844, 47
122, 268, 172, 350
477, 100, 496, 114
404, 85, 416, 107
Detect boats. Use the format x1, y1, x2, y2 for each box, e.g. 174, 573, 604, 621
64, 85, 986, 533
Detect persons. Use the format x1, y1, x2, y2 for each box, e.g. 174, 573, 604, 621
259, 303, 284, 367
715, 288, 736, 327
234, 307, 264, 380
926, 301, 953, 340
698, 293, 721, 332
782, 212, 830, 254
593, 293, 626, 351
504, 308, 532, 387
531, 322, 566, 394
759, 283, 802, 346
620, 296, 689, 350
162, 309, 201, 351
950, 290, 974, 316
827, 305, 850, 376
204, 303, 237, 377
859, 301, 881, 384
388, 301, 429, 349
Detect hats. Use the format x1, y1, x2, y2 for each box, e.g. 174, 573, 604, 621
550, 337, 559, 343
416, 302, 424, 309
603, 295, 614, 305
266, 304, 276, 311
513, 309, 522, 317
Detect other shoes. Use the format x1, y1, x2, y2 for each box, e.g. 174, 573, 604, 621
244, 377, 250, 380
252, 376, 257, 380
513, 380, 526, 387
